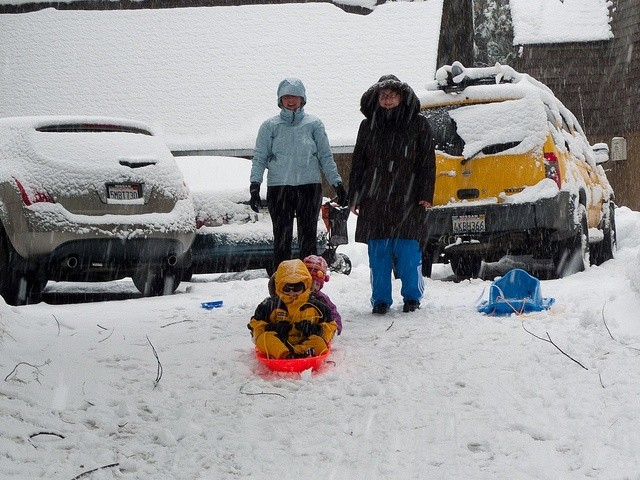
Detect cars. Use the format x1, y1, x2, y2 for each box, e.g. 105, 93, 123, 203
177, 155, 336, 275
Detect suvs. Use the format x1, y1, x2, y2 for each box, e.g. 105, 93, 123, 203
391, 60, 617, 281
2, 112, 197, 306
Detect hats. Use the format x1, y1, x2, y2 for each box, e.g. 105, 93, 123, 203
303, 255, 330, 291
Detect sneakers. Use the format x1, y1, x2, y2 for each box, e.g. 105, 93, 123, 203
372, 304, 388, 313
294, 348, 316, 358
403, 300, 420, 312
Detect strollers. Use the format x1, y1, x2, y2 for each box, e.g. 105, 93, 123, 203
318, 194, 352, 276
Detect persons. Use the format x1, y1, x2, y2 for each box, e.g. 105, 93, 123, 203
247, 255, 342, 359
250, 79, 347, 273
348, 75, 436, 315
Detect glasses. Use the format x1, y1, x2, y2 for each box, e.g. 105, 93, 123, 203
380, 91, 400, 98
283, 286, 303, 292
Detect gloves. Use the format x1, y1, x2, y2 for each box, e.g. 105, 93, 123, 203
249, 183, 262, 213
265, 321, 292, 338
333, 185, 347, 207
294, 320, 322, 336
